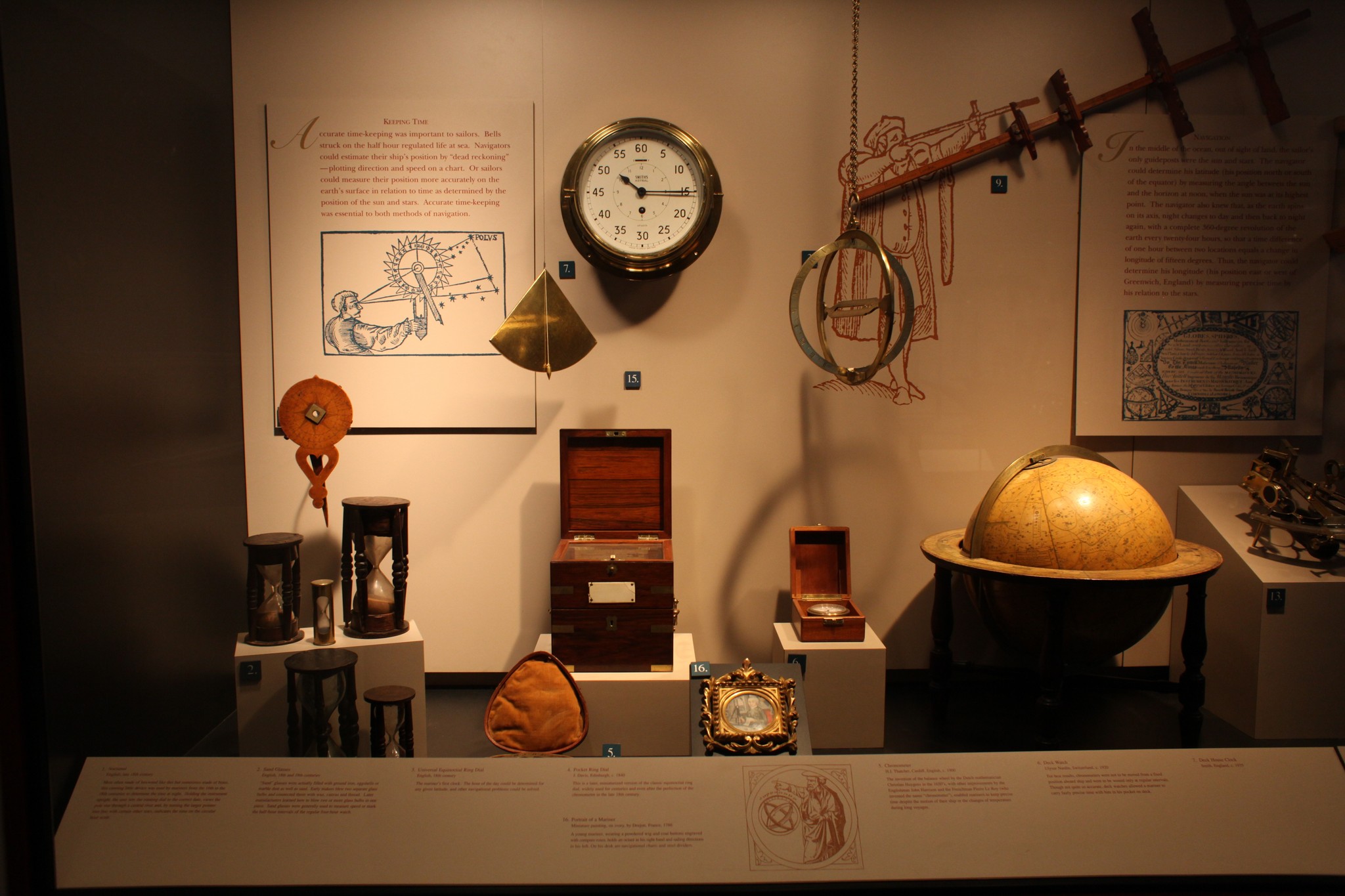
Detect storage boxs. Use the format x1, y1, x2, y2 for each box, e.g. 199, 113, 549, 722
546, 418, 680, 673
788, 520, 871, 642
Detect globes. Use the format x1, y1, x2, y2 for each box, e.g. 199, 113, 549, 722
919, 444, 1225, 753
